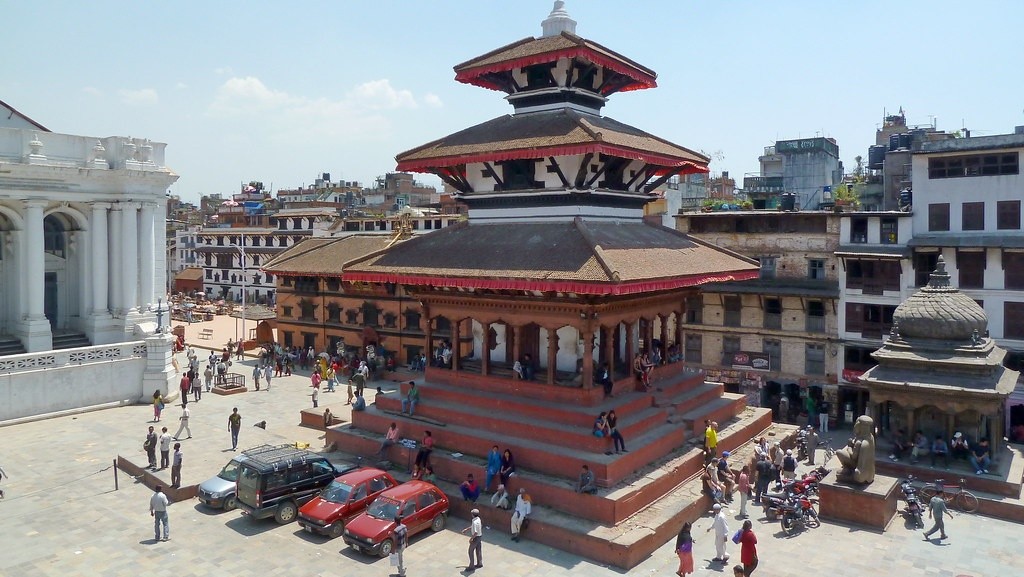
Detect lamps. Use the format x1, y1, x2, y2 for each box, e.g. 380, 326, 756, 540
579, 312, 598, 319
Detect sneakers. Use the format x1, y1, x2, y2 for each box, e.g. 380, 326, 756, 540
984, 469, 989, 474
976, 470, 982, 475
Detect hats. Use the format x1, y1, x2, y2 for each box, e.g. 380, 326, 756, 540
470, 509, 479, 515
954, 432, 963, 438
713, 503, 721, 509
786, 448, 793, 456
496, 484, 505, 490
154, 484, 164, 492
773, 442, 781, 446
721, 450, 729, 458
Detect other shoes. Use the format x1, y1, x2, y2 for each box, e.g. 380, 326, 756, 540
922, 532, 929, 540
721, 555, 729, 563
710, 491, 734, 508
941, 534, 948, 539
889, 454, 895, 459
893, 458, 899, 462
465, 567, 475, 571
163, 537, 171, 542
155, 537, 162, 542
712, 557, 722, 561
146, 464, 170, 472
175, 484, 180, 489
169, 484, 176, 488
474, 565, 484, 568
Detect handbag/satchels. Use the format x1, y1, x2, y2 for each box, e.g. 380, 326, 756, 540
752, 555, 758, 565
732, 529, 743, 544
143, 440, 151, 452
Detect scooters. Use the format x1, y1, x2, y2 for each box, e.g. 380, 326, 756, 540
793, 427, 818, 460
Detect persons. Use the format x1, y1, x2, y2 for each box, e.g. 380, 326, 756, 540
574, 465, 598, 494
499, 449, 514, 487
391, 516, 409, 577
187, 308, 192, 324
634, 338, 684, 390
150, 485, 171, 541
144, 336, 452, 488
519, 354, 536, 382
462, 508, 483, 571
593, 410, 629, 454
889, 423, 1024, 474
460, 474, 481, 502
483, 445, 502, 491
702, 392, 830, 577
923, 489, 953, 539
594, 362, 614, 398
510, 487, 532, 543
490, 485, 513, 510
674, 522, 696, 577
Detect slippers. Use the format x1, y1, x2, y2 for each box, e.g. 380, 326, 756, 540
676, 571, 683, 577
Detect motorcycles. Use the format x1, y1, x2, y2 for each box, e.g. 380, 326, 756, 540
898, 474, 927, 529
760, 465, 832, 536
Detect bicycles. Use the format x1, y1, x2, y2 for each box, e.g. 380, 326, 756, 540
917, 478, 979, 514
819, 437, 836, 467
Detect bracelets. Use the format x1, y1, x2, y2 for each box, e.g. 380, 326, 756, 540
912, 443, 913, 447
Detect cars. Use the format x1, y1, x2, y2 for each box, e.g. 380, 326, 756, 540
297, 466, 400, 539
197, 449, 270, 511
342, 478, 450, 557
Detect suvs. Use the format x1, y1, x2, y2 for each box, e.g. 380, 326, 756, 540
234, 443, 361, 526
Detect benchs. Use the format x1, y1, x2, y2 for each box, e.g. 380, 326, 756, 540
199, 329, 214, 340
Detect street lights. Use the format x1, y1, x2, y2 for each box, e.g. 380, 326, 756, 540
228, 233, 247, 343
